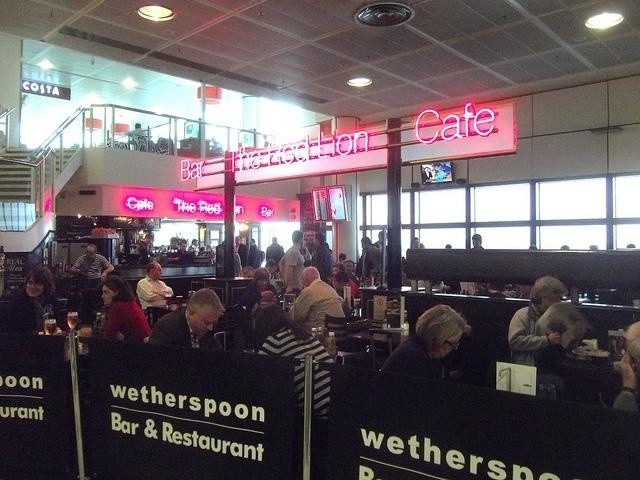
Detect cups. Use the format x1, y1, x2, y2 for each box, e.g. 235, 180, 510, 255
44, 319, 57, 336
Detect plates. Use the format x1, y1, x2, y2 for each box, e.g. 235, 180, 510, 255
572, 347, 609, 358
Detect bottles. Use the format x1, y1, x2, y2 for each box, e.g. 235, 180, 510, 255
311, 327, 318, 339
327, 332, 337, 356
318, 327, 325, 344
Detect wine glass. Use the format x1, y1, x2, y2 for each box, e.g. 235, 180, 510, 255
66, 311, 79, 331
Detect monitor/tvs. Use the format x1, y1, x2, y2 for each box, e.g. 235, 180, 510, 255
420, 162, 455, 185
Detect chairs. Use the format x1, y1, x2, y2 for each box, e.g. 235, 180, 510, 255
0, 277, 640, 454
107, 133, 174, 155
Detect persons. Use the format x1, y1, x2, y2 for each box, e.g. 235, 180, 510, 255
130, 123, 156, 150
0, 232, 638, 411
